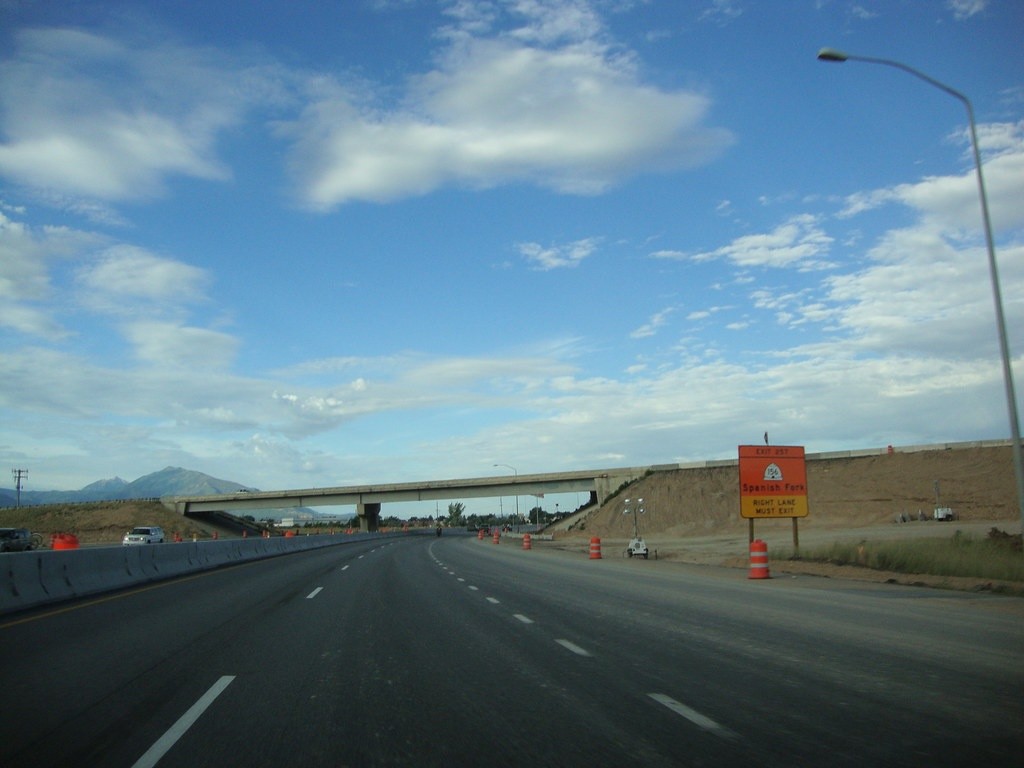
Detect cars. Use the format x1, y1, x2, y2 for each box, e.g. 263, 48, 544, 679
467, 523, 478, 531
502, 523, 512, 531
235, 488, 251, 495
478, 523, 490, 534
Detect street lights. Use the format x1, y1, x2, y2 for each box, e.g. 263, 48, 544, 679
817, 48, 1024, 542
494, 464, 519, 514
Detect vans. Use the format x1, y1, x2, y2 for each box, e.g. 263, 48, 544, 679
0, 528, 33, 550
122, 526, 165, 545
